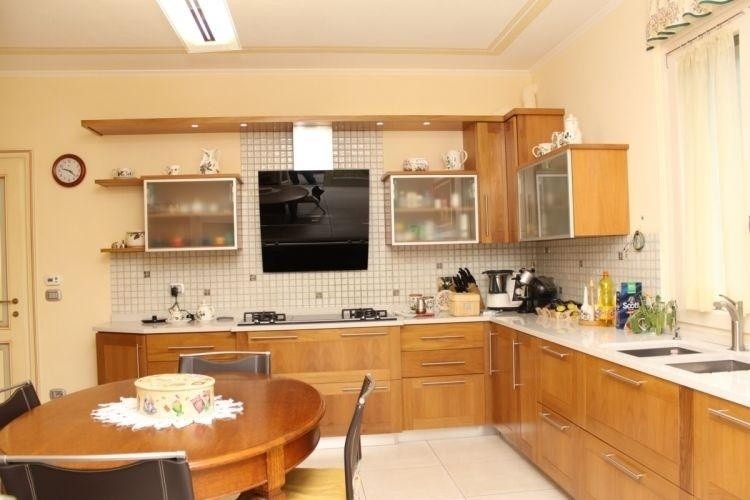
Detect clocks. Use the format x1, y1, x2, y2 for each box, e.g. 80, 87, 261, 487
52, 154, 87, 188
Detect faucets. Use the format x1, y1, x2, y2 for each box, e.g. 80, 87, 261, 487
712, 294, 744, 351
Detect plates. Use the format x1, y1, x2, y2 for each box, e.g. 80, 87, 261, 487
113, 176, 138, 179
166, 319, 191, 324
436, 290, 453, 312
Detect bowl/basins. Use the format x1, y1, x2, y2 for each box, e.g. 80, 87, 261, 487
133, 373, 216, 416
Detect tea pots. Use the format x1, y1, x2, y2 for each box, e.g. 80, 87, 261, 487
199, 146, 221, 174
195, 300, 215, 322
441, 149, 468, 170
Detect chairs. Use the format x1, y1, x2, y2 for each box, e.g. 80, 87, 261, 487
178, 350, 271, 375
0, 380, 41, 436
0, 451, 195, 500
235, 371, 374, 499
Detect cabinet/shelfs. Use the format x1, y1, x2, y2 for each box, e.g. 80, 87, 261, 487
96, 327, 144, 386
463, 114, 509, 244
517, 142, 630, 241
93, 171, 244, 252
244, 320, 401, 436
401, 319, 488, 432
384, 175, 480, 245
488, 317, 542, 464
578, 352, 681, 499
504, 108, 565, 243
142, 179, 241, 252
144, 331, 244, 377
681, 383, 749, 500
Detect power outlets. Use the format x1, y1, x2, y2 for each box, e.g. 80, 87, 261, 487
533, 335, 585, 499
171, 283, 184, 294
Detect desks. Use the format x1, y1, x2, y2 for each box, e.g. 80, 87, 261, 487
0, 374, 326, 500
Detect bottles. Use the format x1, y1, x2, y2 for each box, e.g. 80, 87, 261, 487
408, 294, 434, 316
552, 113, 583, 146
581, 272, 615, 328
397, 188, 422, 209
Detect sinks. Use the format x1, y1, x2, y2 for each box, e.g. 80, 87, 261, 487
666, 359, 750, 375
617, 346, 702, 357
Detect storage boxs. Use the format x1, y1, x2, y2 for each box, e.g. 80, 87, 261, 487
447, 293, 481, 317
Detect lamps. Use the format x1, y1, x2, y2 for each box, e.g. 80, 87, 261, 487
157, 0, 243, 55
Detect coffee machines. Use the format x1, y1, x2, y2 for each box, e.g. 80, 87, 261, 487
484, 269, 524, 309
517, 267, 556, 314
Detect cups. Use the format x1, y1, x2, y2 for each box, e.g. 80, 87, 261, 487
126, 231, 144, 247
402, 159, 429, 171
168, 165, 180, 175
532, 142, 555, 157
117, 168, 133, 176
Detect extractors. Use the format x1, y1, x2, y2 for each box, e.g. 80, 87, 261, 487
257, 122, 370, 273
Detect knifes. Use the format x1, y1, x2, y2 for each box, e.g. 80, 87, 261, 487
454, 267, 477, 294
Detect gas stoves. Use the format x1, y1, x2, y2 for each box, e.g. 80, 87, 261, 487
237, 309, 398, 325
